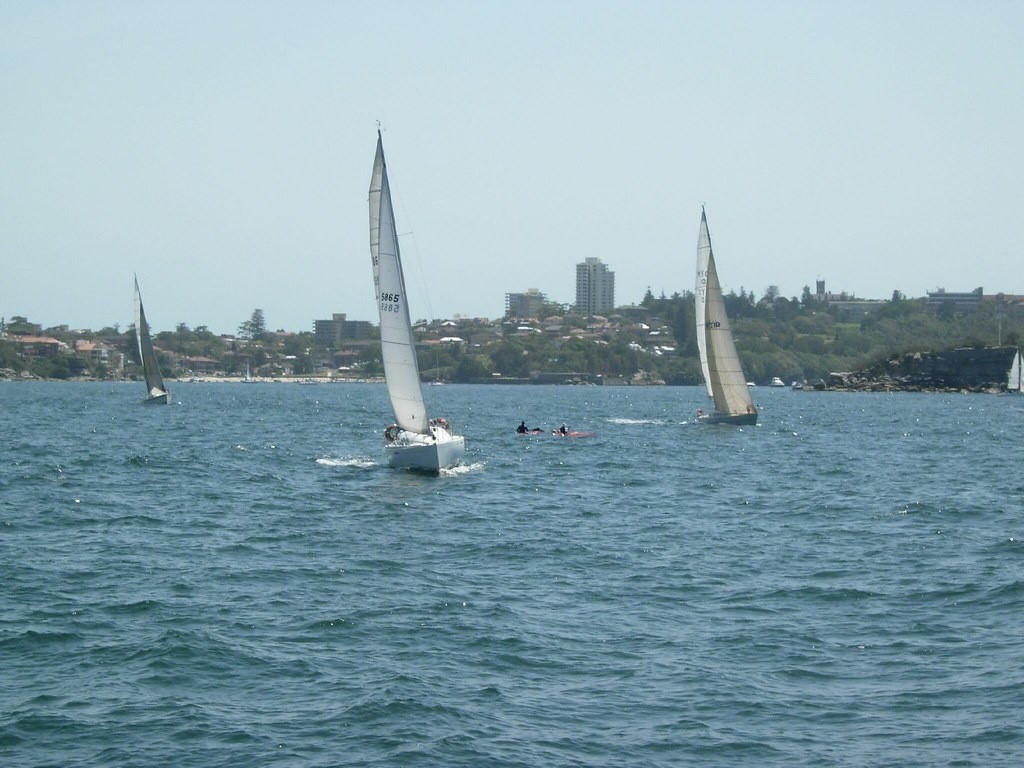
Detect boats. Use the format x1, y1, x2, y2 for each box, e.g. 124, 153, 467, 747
791, 381, 802, 389
771, 377, 784, 387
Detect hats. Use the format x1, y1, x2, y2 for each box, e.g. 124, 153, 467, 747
433, 420, 437, 424
561, 422, 566, 427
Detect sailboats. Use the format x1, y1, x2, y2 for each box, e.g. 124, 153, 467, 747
998, 349, 1024, 398
690, 207, 760, 424
365, 129, 463, 473
131, 274, 169, 405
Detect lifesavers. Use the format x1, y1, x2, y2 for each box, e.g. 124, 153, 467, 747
433, 419, 447, 429
746, 405, 755, 414
385, 424, 399, 442
697, 409, 702, 417
430, 419, 434, 427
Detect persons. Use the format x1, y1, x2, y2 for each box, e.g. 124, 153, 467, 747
559, 423, 567, 434
516, 421, 528, 433
697, 407, 702, 416
